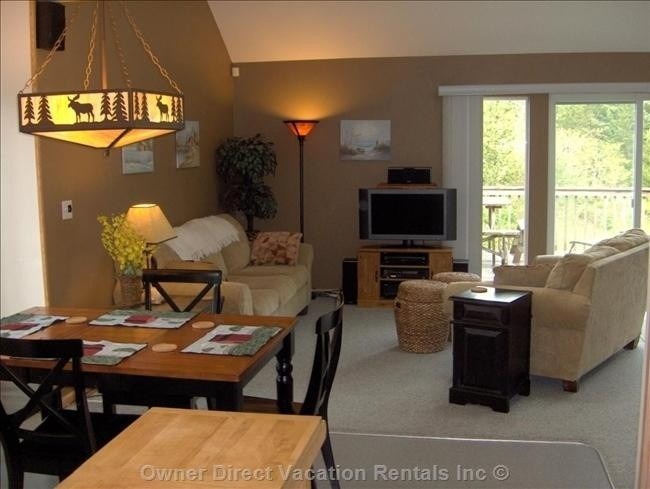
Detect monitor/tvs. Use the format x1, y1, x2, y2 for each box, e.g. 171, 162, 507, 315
367, 189, 447, 249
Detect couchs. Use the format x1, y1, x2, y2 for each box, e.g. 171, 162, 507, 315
147, 213, 313, 316
442, 228, 650, 393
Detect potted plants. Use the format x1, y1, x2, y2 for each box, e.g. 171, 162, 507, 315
97, 211, 146, 308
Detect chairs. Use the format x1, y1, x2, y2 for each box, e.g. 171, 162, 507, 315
215, 291, 344, 489
97, 268, 221, 414
0, 337, 143, 489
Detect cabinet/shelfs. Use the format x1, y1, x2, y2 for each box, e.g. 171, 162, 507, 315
448, 287, 532, 413
357, 243, 453, 307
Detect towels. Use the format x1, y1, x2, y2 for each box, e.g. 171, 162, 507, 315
162, 214, 240, 261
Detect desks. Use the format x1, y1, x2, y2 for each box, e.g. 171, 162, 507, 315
482, 230, 519, 265
109, 294, 224, 315
52, 407, 328, 489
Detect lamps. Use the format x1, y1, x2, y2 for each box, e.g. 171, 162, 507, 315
283, 119, 318, 241
126, 203, 178, 310
17, 0, 184, 158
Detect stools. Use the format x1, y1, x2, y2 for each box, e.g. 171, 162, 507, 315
432, 271, 480, 340
509, 222, 524, 264
394, 279, 450, 353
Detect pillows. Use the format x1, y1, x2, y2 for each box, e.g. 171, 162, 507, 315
492, 263, 555, 287
594, 227, 650, 253
251, 230, 303, 264
543, 247, 622, 293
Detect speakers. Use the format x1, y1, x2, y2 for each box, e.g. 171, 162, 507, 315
453, 259, 469, 272
342, 258, 357, 305
37, 1, 66, 51
